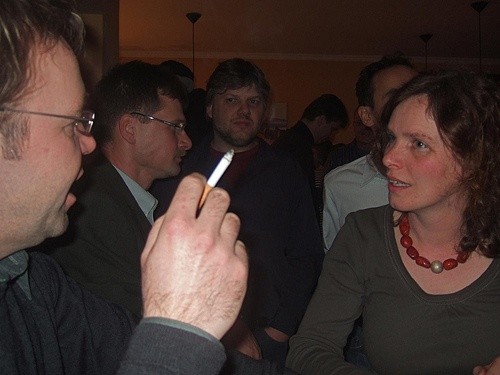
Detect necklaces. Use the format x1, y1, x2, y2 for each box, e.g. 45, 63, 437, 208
399, 211, 470, 272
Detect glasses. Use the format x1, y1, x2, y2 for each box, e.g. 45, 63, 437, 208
0, 107, 96, 136
130, 111, 189, 136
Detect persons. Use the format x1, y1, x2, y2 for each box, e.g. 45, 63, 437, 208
28, 59, 262, 360
0, 0, 299, 375
0, 0, 500, 255
284, 63, 500, 375
